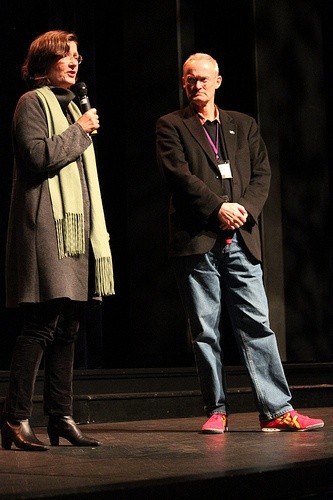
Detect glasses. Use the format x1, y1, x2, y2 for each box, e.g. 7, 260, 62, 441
57, 52, 83, 65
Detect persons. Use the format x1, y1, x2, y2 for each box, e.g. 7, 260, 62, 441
154, 53, 324, 434
0, 31, 115, 450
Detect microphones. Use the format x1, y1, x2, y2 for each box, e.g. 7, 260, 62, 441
73, 81, 98, 136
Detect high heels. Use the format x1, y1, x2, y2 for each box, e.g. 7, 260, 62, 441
47, 414, 103, 447
1, 419, 50, 451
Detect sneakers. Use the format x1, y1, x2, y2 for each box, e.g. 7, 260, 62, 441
260, 409, 324, 432
200, 413, 229, 434
